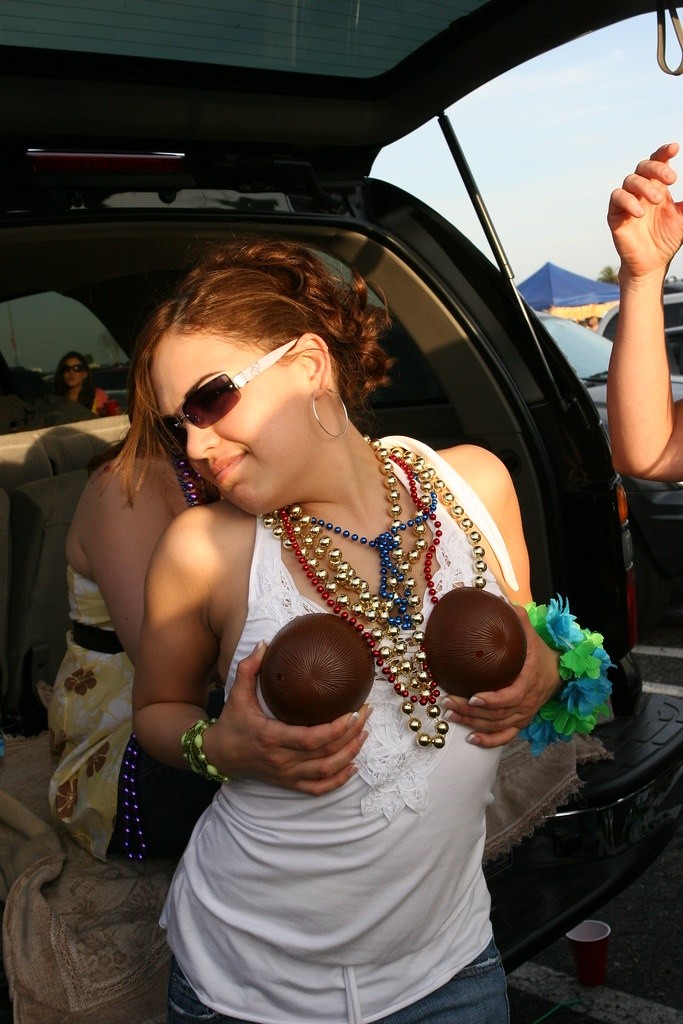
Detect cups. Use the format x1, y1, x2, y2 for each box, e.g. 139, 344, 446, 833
566, 919, 611, 987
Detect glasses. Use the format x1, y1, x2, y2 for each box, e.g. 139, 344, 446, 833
61, 364, 85, 373
155, 340, 298, 453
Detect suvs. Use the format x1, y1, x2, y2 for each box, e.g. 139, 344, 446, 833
1, 0, 683, 1024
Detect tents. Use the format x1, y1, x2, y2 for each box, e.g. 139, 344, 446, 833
517, 262, 620, 321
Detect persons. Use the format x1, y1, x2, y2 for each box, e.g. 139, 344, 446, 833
115, 243, 611, 1023
575, 317, 598, 331
48, 302, 217, 863
608, 143, 683, 477
54, 350, 122, 417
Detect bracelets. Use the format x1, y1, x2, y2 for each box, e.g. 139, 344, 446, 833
181, 717, 235, 782
519, 600, 611, 756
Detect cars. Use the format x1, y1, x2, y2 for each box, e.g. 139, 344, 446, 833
528, 307, 683, 627
593, 291, 683, 380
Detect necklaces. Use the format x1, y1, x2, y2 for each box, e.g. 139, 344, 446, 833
124, 734, 146, 860
256, 435, 487, 747
172, 455, 205, 507
